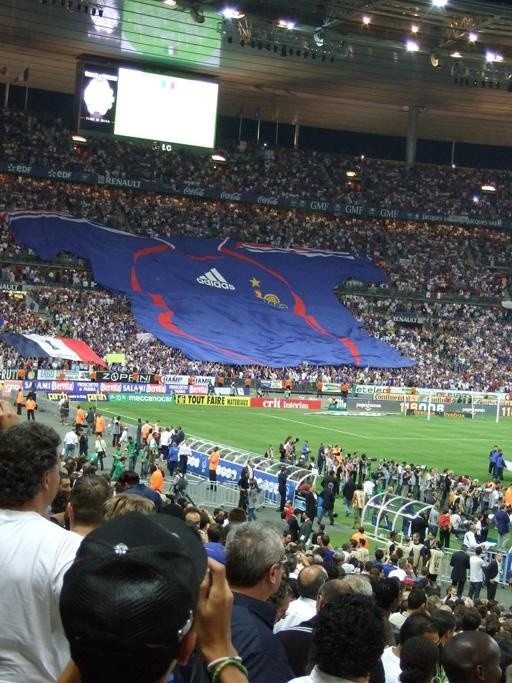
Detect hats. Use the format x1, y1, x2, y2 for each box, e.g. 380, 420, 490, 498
59, 511, 209, 659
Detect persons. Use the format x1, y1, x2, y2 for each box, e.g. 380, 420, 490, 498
0, 111, 512, 682
